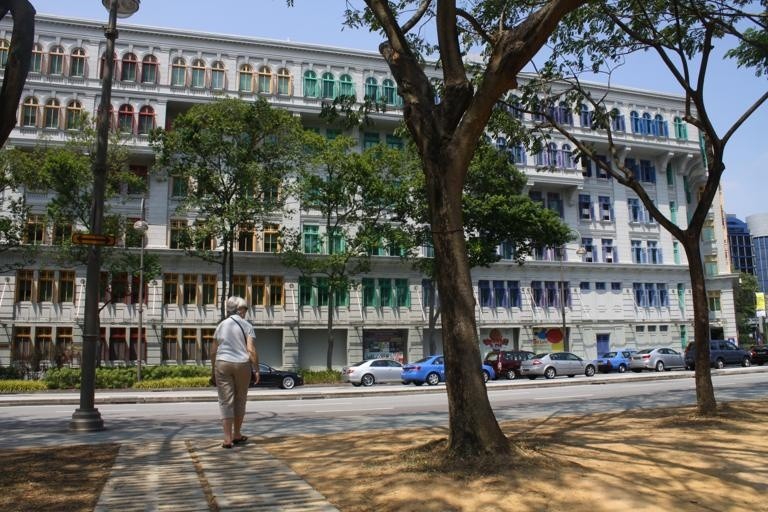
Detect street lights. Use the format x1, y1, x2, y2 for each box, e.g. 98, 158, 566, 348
133, 198, 148, 381
66, 0, 140, 434
555, 229, 587, 352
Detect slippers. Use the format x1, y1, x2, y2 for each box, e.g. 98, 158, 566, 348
223, 435, 248, 448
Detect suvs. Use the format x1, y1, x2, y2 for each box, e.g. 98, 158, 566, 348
483, 350, 537, 380
684, 340, 754, 370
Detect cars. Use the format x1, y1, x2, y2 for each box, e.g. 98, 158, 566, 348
628, 347, 690, 372
519, 352, 596, 380
342, 358, 411, 387
400, 355, 496, 386
593, 350, 637, 374
751, 344, 768, 365
249, 363, 304, 389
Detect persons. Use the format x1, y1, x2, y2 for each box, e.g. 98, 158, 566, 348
212, 295, 261, 450
